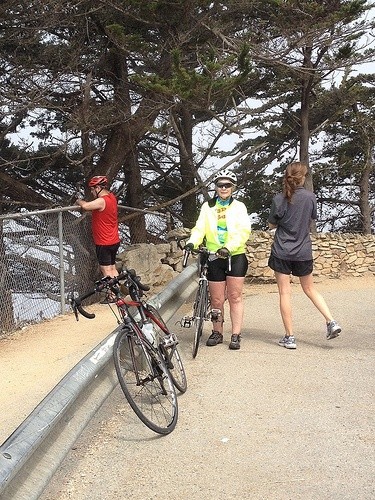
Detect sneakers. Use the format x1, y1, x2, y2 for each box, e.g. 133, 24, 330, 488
229, 332, 242, 349
206, 330, 223, 346
278, 335, 296, 349
326, 321, 341, 340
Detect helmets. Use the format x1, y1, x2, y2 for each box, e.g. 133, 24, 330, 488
212, 168, 238, 188
88, 176, 109, 189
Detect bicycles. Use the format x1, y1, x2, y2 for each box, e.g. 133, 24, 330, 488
66, 269, 187, 435
175, 235, 230, 358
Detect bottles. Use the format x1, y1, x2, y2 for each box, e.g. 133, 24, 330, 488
133, 317, 155, 345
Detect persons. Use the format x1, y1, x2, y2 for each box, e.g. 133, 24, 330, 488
183, 170, 252, 349
73, 175, 122, 305
266, 162, 341, 349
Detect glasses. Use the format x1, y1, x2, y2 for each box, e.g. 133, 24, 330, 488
215, 182, 234, 189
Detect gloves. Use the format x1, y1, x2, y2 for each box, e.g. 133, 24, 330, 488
215, 247, 229, 259
183, 243, 194, 253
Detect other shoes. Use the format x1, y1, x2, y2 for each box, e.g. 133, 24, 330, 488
100, 294, 117, 304
